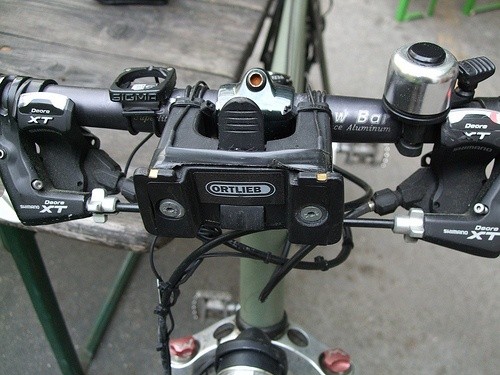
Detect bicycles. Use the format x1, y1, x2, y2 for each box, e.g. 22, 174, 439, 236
1, 1, 500, 375
